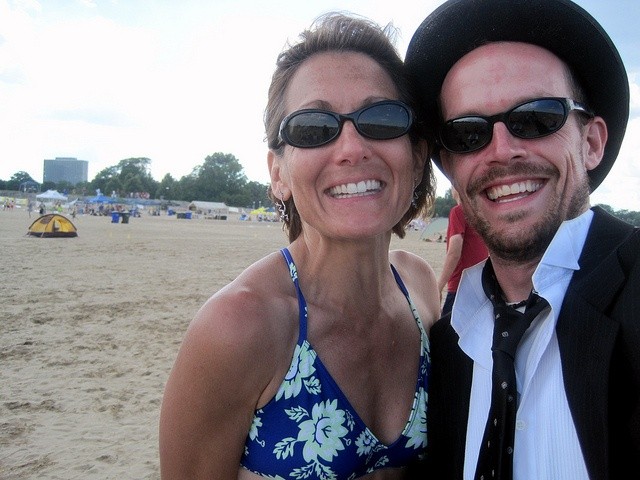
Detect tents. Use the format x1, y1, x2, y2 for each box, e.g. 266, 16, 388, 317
26, 213, 78, 237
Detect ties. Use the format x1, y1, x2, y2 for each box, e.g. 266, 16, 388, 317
472, 256, 550, 480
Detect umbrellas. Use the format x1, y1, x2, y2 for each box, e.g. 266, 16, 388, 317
252, 207, 274, 223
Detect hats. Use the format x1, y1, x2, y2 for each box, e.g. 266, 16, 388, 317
403, 1, 631, 193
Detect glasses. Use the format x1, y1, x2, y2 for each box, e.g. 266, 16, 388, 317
273, 99, 419, 149
432, 95, 586, 155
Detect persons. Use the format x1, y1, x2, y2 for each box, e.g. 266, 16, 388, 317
160, 10, 442, 479
70, 203, 165, 217
437, 183, 491, 318
3, 198, 15, 210
26, 200, 34, 218
402, 0, 640, 480
57, 202, 62, 211
37, 201, 47, 216
